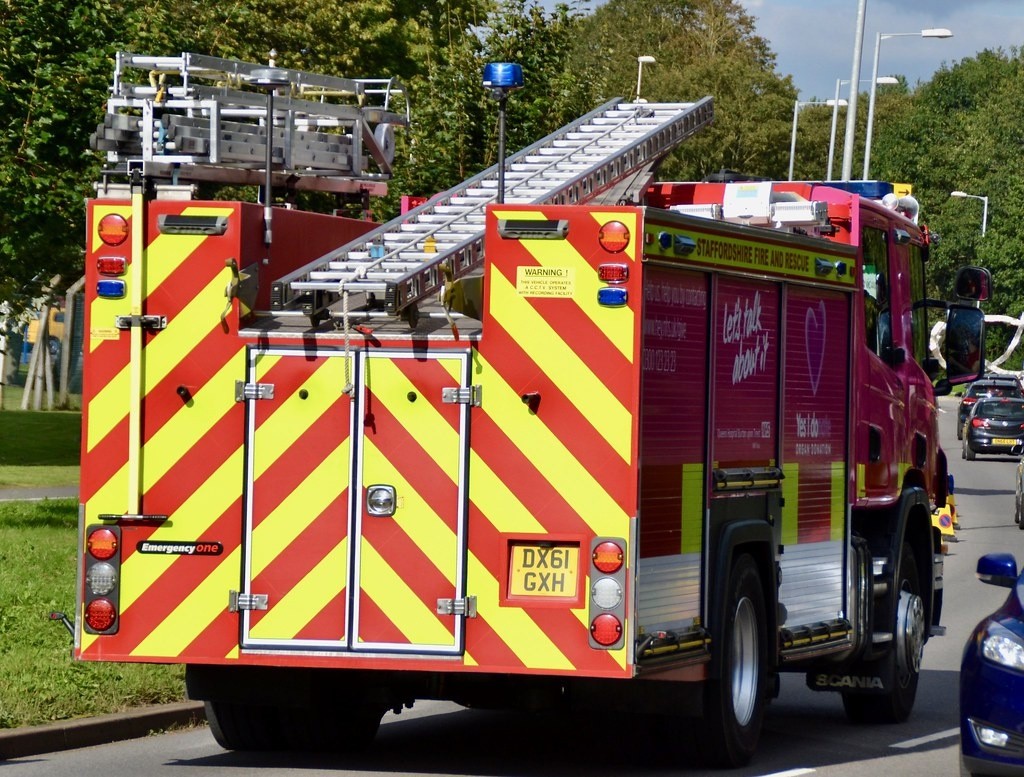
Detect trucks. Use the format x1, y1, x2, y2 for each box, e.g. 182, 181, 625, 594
22, 296, 66, 354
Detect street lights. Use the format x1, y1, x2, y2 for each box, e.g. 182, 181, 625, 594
788, 27, 954, 181
636, 56, 656, 104
951, 191, 989, 308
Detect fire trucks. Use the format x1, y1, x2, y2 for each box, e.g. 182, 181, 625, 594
46, 51, 994, 755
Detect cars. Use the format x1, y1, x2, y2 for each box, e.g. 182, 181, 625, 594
961, 397, 1024, 461
957, 374, 1024, 440
960, 552, 1024, 777
1015, 455, 1024, 530
